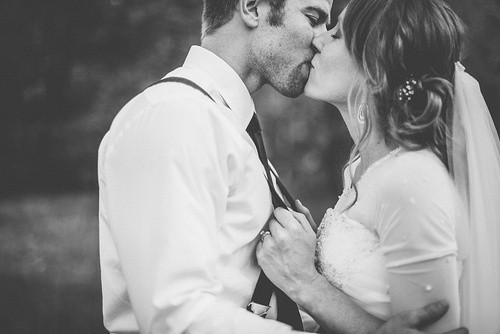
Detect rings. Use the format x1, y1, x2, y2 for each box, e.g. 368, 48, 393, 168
260, 230, 270, 241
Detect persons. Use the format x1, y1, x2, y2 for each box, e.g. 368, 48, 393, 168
98, 0, 466, 333
257, 0, 469, 333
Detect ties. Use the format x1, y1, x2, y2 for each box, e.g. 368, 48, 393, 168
245, 111, 305, 332
